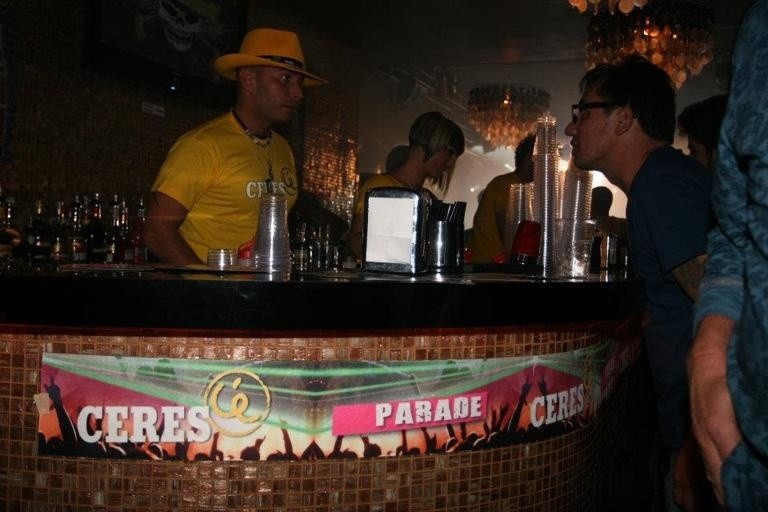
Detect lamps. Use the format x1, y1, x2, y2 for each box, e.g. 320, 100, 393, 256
566, 0, 670, 56
583, 12, 716, 94
469, 84, 552, 150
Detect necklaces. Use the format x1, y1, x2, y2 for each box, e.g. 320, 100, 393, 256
234, 111, 276, 179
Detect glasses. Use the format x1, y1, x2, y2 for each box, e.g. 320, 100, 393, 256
571, 101, 624, 120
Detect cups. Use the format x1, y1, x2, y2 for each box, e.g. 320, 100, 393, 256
251, 192, 294, 274
535, 115, 562, 278
561, 168, 593, 278
507, 182, 538, 259
207, 246, 237, 267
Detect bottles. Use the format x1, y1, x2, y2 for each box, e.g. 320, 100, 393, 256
70, 197, 88, 262
334, 247, 344, 270
106, 194, 124, 264
83, 197, 94, 263
32, 200, 50, 266
121, 203, 134, 262
314, 231, 322, 272
131, 205, 146, 265
319, 227, 327, 271
325, 226, 333, 270
295, 231, 307, 273
88, 194, 106, 262
305, 231, 318, 272
51, 201, 72, 264
3, 201, 20, 266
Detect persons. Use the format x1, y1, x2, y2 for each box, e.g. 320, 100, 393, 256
591, 186, 625, 235
351, 112, 465, 260
37, 364, 581, 462
566, 53, 714, 511
690, 1, 768, 512
473, 134, 535, 264
143, 27, 329, 265
679, 93, 729, 167
384, 144, 410, 173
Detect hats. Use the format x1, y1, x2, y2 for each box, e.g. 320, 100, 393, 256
216, 28, 331, 90
